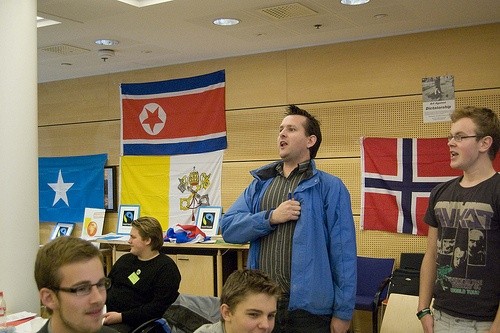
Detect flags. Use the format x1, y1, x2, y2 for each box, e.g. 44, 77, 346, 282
120, 150, 223, 236
119, 69, 228, 156
38, 153, 108, 222
360, 137, 500, 235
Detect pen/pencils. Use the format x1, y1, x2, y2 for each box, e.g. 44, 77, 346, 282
288, 187, 292, 201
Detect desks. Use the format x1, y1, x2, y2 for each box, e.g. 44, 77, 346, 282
96, 233, 249, 298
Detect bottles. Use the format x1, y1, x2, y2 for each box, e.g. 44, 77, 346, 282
0, 291, 7, 329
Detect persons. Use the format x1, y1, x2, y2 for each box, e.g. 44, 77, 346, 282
417, 107, 500, 333
220, 105, 357, 333
99, 217, 181, 333
192, 269, 278, 333
35, 236, 121, 333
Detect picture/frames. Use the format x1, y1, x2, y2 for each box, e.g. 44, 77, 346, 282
49, 222, 76, 241
196, 206, 222, 235
116, 205, 140, 235
104, 165, 118, 213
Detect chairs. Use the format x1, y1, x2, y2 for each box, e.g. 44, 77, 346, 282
354, 256, 395, 333
130, 293, 181, 333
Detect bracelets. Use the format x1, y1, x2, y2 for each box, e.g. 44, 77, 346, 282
415, 308, 430, 320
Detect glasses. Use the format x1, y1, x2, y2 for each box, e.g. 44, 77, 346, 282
48, 278, 112, 295
448, 134, 486, 143
